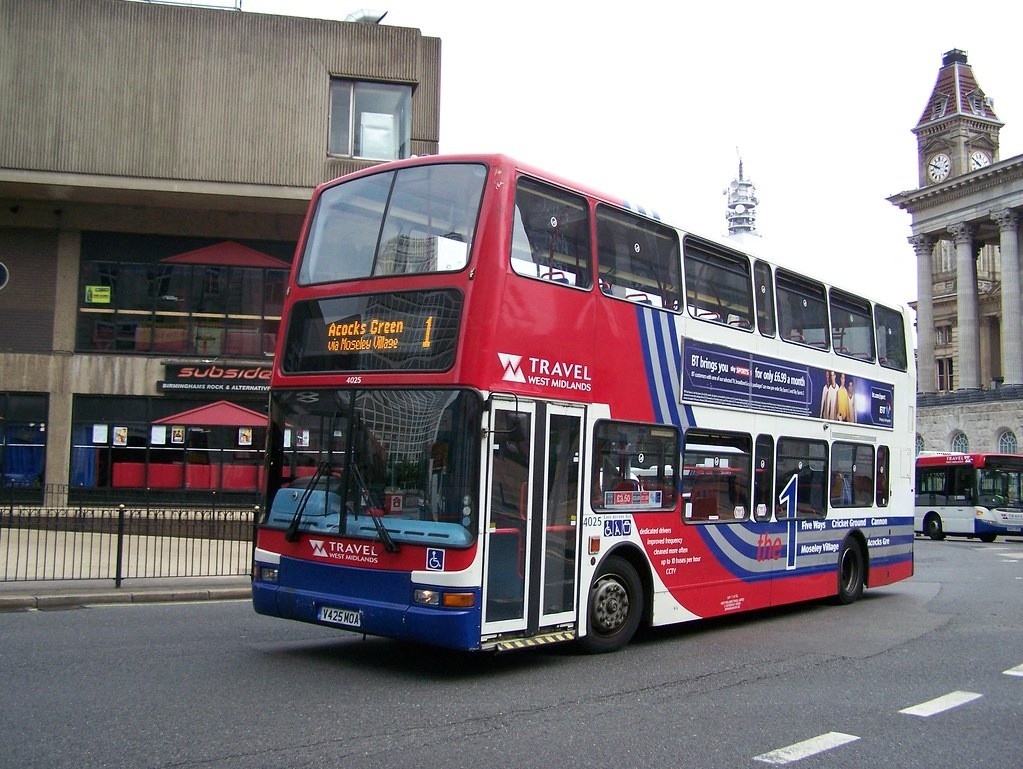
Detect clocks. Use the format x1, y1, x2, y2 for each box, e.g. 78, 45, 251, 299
969, 149, 993, 173
928, 153, 951, 183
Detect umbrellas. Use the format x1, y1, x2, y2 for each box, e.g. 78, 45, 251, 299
156, 238, 294, 271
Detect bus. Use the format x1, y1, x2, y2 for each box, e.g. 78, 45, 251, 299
236, 151, 919, 656
915, 450, 1023, 542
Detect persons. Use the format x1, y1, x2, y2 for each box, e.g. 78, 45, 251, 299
599, 435, 854, 519
819, 370, 858, 424
